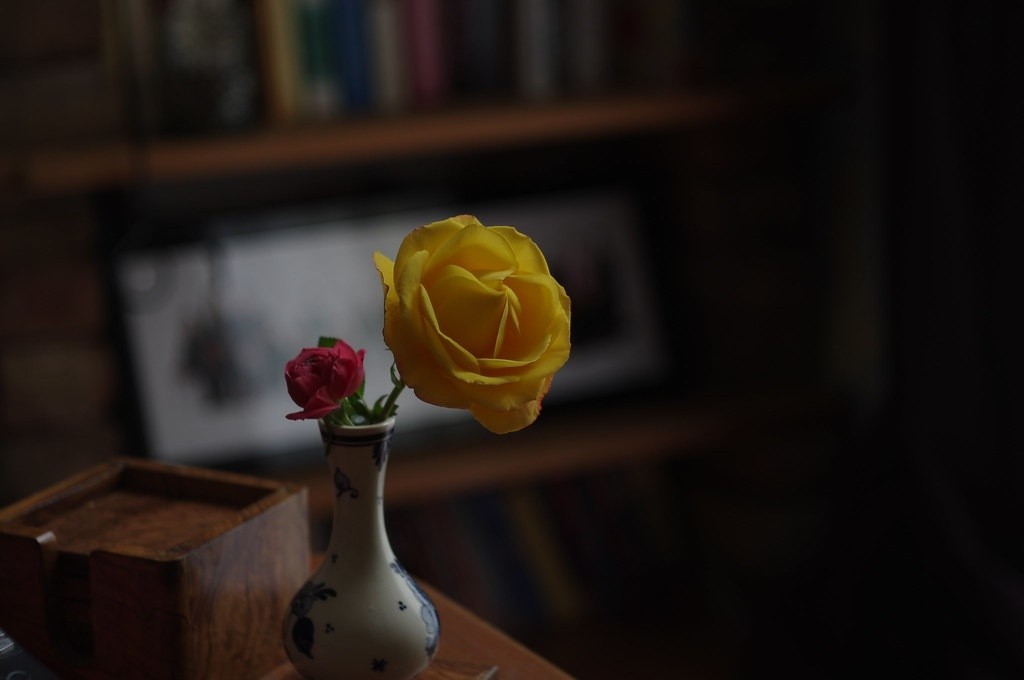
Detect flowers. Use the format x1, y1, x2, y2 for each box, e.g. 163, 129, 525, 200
285, 215, 573, 434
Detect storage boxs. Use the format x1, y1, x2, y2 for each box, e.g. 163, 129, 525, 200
0, 457, 312, 680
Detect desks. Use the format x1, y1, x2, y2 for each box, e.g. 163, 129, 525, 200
313, 555, 575, 680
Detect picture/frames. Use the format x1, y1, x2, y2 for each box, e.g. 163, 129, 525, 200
99, 174, 690, 474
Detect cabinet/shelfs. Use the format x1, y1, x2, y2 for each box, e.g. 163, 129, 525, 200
0, 73, 805, 680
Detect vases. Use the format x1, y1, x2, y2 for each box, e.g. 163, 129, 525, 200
281, 409, 441, 680
